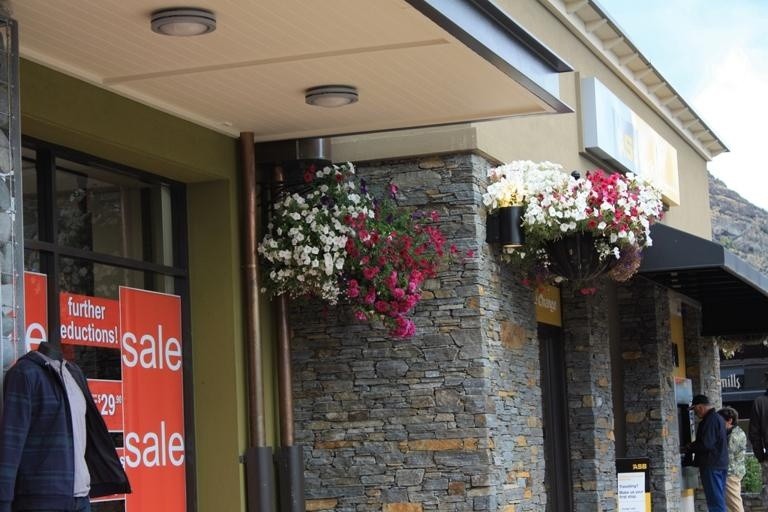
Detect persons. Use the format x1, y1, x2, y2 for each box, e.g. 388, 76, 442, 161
0, 341, 132, 512
749, 371, 768, 511
687, 394, 731, 511
716, 406, 749, 511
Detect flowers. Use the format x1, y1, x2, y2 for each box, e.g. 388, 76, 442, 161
258, 160, 476, 340
484, 160, 664, 296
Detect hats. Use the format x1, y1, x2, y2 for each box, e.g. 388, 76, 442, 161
687, 394, 710, 411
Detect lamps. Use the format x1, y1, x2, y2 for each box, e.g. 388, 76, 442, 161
305, 86, 359, 108
150, 10, 216, 36
